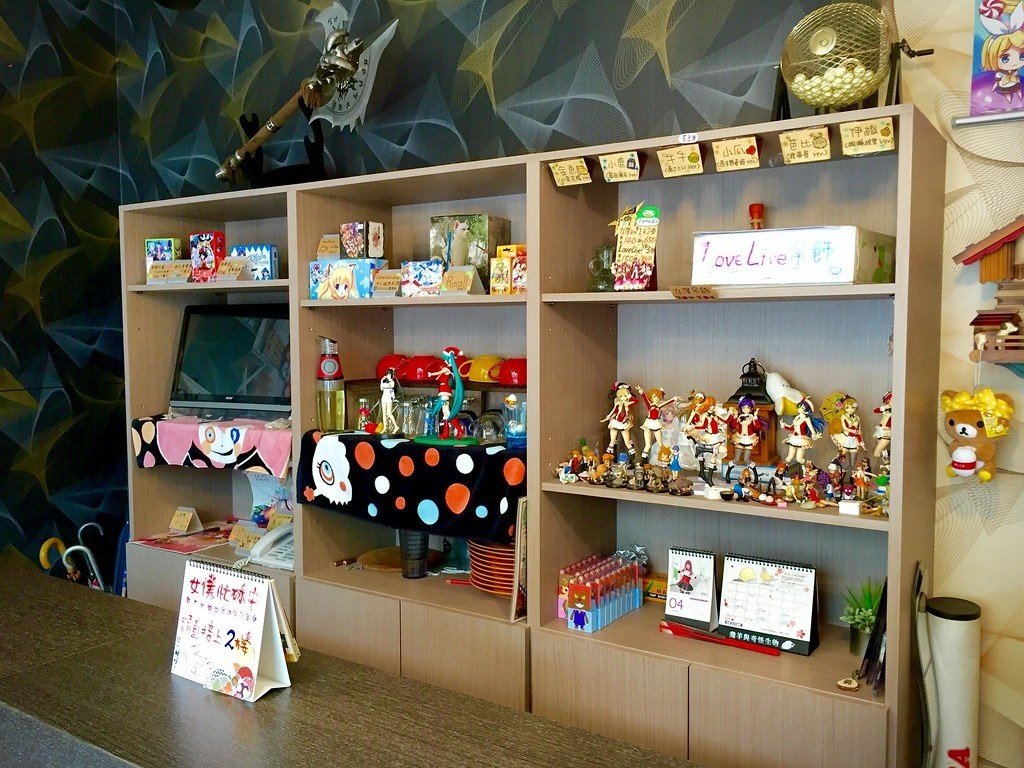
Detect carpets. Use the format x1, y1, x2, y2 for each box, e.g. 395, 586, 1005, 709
0, 701, 142, 768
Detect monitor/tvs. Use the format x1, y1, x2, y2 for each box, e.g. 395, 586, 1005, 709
169, 304, 290, 419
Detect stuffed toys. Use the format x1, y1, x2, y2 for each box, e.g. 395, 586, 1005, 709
940, 390, 1015, 484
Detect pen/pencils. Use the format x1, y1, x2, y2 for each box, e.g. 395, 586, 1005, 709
445, 578, 473, 585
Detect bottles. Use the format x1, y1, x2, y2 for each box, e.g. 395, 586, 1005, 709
315, 336, 345, 433
585, 245, 615, 292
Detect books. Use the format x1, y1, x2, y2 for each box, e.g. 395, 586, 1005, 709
511, 497, 528, 627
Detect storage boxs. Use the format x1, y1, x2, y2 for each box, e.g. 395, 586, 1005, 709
189, 232, 227, 282
558, 553, 668, 633
145, 238, 181, 274
230, 243, 279, 280
430, 215, 527, 295
692, 225, 897, 287
309, 221, 388, 300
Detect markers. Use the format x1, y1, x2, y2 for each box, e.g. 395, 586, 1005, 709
331, 558, 357, 567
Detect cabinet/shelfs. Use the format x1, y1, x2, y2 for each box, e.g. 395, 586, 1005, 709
118, 103, 947, 768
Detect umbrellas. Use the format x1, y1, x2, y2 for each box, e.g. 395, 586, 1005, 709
39, 522, 129, 599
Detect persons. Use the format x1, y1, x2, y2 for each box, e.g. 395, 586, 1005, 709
554, 382, 892, 516
428, 347, 464, 440
380, 367, 400, 434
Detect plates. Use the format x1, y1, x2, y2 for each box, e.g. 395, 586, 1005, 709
466, 538, 516, 600
357, 545, 443, 572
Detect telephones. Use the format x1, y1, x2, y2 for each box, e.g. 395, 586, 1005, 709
248, 521, 295, 572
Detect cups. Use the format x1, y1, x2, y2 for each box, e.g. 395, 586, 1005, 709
355, 389, 474, 437
398, 529, 430, 579
